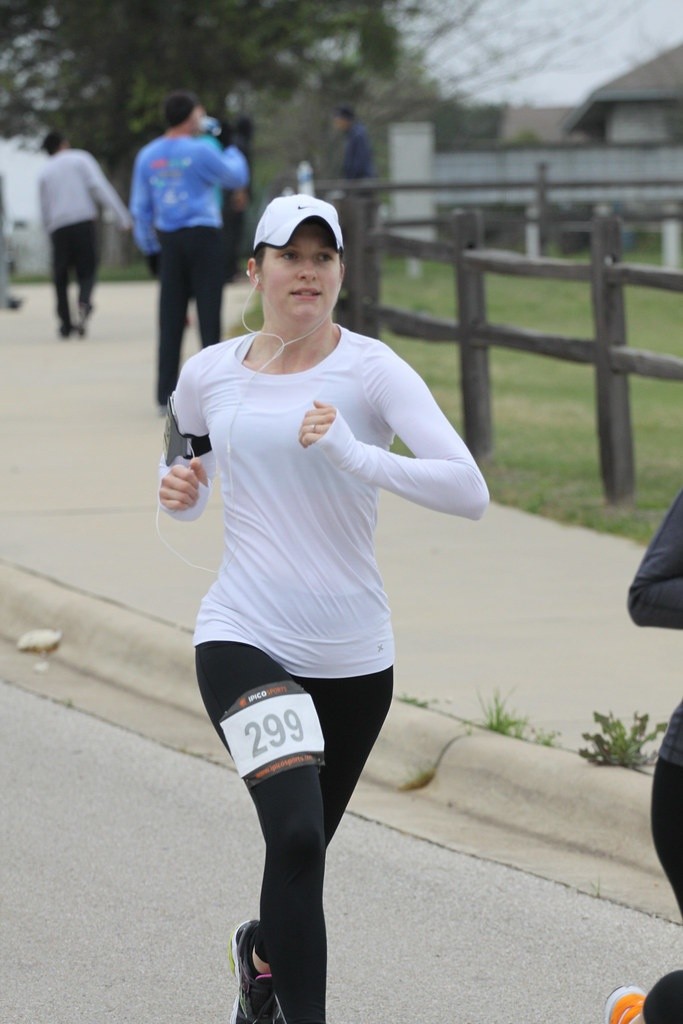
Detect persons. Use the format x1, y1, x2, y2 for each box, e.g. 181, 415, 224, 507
39, 128, 131, 334
334, 101, 380, 224
155, 194, 490, 1024
130, 97, 256, 414
602, 482, 683, 1024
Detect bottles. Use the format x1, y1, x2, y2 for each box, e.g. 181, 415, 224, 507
296, 162, 315, 198
199, 114, 222, 137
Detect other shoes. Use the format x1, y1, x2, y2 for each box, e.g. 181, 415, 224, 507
605, 987, 647, 1023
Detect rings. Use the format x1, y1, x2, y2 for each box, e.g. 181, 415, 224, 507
311, 424, 318, 432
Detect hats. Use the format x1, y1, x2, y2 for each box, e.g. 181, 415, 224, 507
254, 194, 343, 253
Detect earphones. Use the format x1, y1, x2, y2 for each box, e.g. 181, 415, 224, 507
253, 273, 263, 284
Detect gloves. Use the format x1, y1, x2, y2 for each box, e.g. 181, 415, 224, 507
214, 120, 232, 150
147, 252, 161, 275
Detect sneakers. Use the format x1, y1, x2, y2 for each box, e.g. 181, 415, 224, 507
231, 920, 286, 1024
